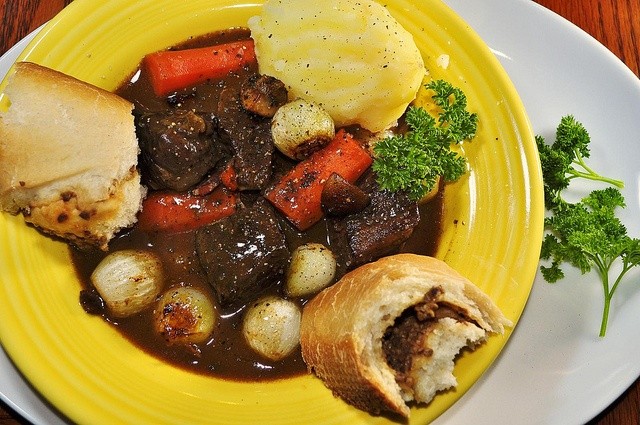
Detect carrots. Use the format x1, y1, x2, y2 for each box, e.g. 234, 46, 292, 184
137, 190, 236, 231
143, 41, 257, 94
266, 127, 374, 231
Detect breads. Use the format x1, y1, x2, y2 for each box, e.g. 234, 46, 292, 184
1, 62, 147, 247
300, 254, 512, 423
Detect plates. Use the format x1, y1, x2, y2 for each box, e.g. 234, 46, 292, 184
0, 0, 640, 424
0, 0, 542, 425
